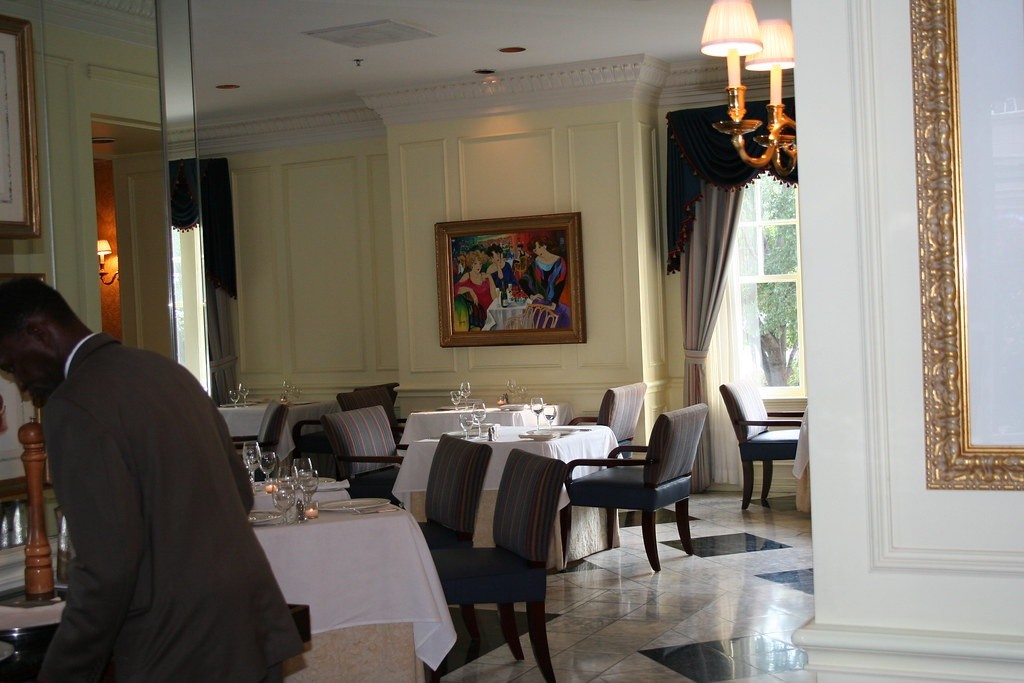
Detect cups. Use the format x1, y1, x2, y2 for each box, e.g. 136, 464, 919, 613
304, 500, 319, 519
496, 393, 505, 404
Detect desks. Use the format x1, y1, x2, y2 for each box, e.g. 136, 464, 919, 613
216, 397, 342, 462
251, 479, 352, 510
251, 503, 457, 683
391, 425, 622, 571
399, 403, 573, 445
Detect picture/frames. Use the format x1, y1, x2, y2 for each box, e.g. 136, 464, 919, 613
0, 12, 42, 240
434, 212, 587, 348
909, 0, 1024, 492
0, 272, 51, 502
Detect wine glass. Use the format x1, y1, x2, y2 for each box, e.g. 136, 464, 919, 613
260, 451, 277, 482
530, 397, 543, 430
239, 383, 249, 407
242, 441, 261, 483
505, 375, 528, 404
450, 391, 460, 411
460, 382, 471, 411
297, 469, 319, 503
280, 376, 304, 402
543, 407, 557, 428
472, 402, 487, 440
459, 413, 474, 441
271, 477, 296, 526
229, 390, 240, 405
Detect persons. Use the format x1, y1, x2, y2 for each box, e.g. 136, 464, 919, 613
0, 277, 303, 683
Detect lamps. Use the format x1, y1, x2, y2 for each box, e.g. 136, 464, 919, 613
701, 0, 797, 177
97, 240, 120, 285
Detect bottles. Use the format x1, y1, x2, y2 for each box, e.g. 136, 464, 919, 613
488, 425, 497, 441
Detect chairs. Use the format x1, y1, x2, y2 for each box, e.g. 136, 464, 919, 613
252, 382, 809, 683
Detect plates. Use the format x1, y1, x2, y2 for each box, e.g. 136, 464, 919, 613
443, 431, 479, 438
497, 403, 525, 410
318, 498, 391, 511
438, 406, 465, 410
319, 477, 336, 484
527, 429, 579, 435
219, 403, 256, 407
248, 509, 283, 525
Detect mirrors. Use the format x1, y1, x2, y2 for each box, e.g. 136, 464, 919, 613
155, 0, 211, 396
